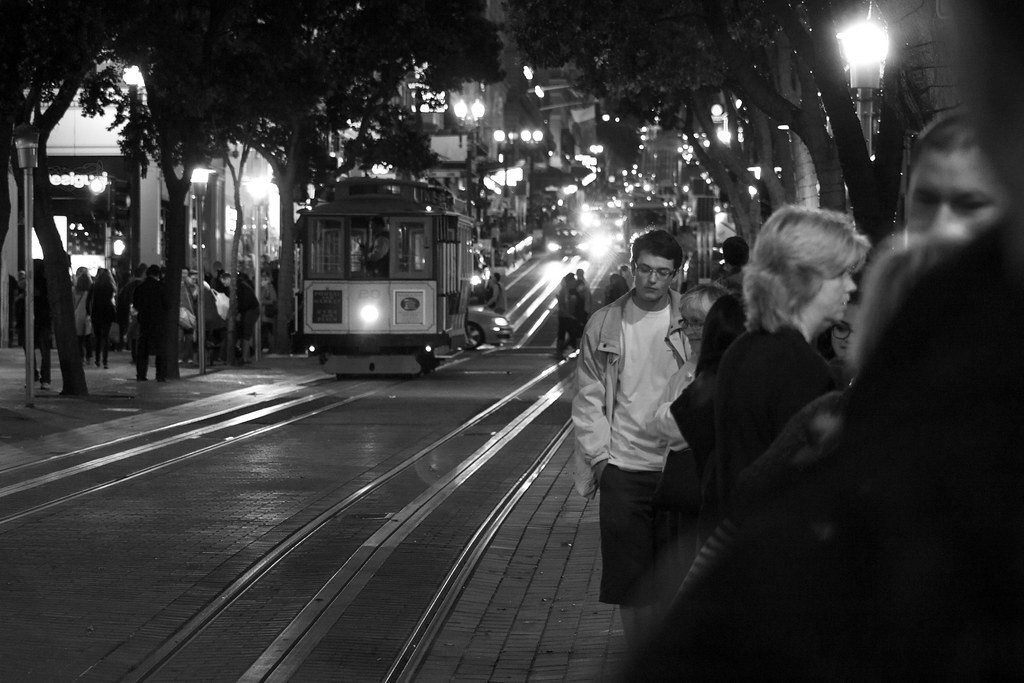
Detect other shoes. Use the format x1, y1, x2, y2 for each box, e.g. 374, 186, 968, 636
40, 382, 50, 389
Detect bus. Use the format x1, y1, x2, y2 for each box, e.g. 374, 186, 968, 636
287, 175, 482, 382
627, 200, 673, 252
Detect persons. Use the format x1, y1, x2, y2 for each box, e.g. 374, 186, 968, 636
71, 263, 166, 382
627, 102, 1024, 683
605, 266, 632, 306
556, 269, 592, 354
475, 273, 507, 314
178, 256, 279, 361
9, 259, 53, 390
571, 232, 694, 628
361, 232, 389, 276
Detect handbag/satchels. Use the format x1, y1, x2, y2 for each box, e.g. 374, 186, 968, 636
179, 305, 197, 330
85, 314, 92, 335
212, 289, 230, 320
109, 322, 121, 343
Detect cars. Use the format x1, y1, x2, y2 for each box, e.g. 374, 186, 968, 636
465, 302, 513, 351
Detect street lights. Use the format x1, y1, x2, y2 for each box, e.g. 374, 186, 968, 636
494, 129, 517, 249
521, 128, 544, 234
13, 122, 44, 407
189, 167, 211, 377
837, 17, 889, 161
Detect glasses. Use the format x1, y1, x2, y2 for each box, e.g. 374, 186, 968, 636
832, 324, 855, 339
634, 262, 676, 281
678, 319, 704, 330
